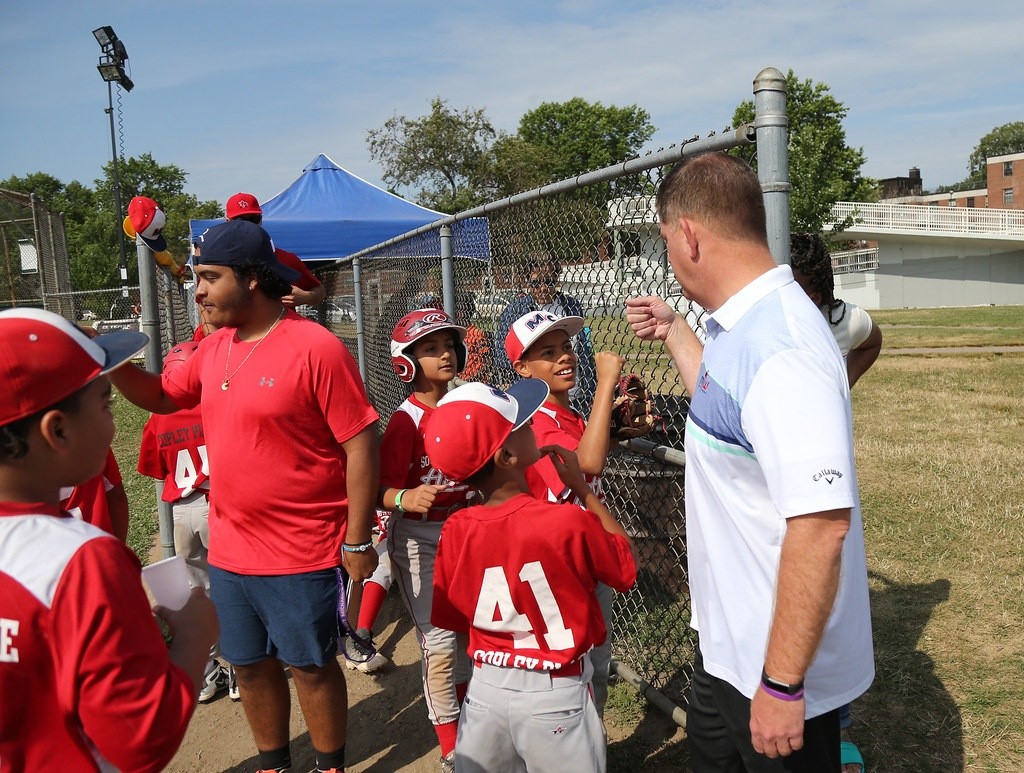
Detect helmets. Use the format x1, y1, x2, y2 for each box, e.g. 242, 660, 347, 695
391, 308, 468, 382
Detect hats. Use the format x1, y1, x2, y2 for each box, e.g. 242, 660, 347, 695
424, 378, 550, 481
192, 219, 301, 282
225, 193, 262, 219
504, 311, 584, 364
0, 308, 150, 426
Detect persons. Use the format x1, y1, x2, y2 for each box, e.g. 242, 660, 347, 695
454, 291, 492, 384
492, 249, 597, 418
373, 308, 493, 767
784, 229, 884, 772
0, 309, 223, 772
224, 192, 326, 312
84, 220, 380, 773
341, 508, 397, 672
626, 151, 880, 773
134, 341, 241, 702
503, 309, 622, 725
373, 276, 419, 384
426, 375, 637, 773
58, 446, 131, 545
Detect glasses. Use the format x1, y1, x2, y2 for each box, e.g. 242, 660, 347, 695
235, 216, 262, 225
530, 278, 556, 287
524, 417, 534, 428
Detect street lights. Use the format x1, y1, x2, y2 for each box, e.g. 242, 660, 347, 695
93, 25, 130, 322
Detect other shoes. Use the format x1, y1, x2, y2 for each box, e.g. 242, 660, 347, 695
309, 757, 344, 773
152, 611, 176, 646
255, 756, 293, 773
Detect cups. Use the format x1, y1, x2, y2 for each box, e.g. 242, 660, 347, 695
141, 553, 192, 613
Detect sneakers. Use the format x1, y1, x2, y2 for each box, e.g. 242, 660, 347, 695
346, 628, 388, 673
229, 664, 241, 702
439, 749, 455, 773
197, 660, 230, 703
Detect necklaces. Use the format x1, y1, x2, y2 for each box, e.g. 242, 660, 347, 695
222, 308, 284, 392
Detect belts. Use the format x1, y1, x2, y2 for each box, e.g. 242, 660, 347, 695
474, 658, 584, 678
402, 507, 465, 522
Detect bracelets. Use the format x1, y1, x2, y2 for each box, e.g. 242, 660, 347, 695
760, 666, 805, 694
341, 542, 373, 561
394, 488, 407, 512
345, 538, 371, 547
760, 681, 804, 701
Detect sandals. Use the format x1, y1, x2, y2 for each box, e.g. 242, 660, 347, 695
840, 742, 864, 773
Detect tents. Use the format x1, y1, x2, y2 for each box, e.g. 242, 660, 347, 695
188, 151, 496, 331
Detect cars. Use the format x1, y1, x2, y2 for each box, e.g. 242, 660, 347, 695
295, 291, 516, 324
75, 309, 98, 321
